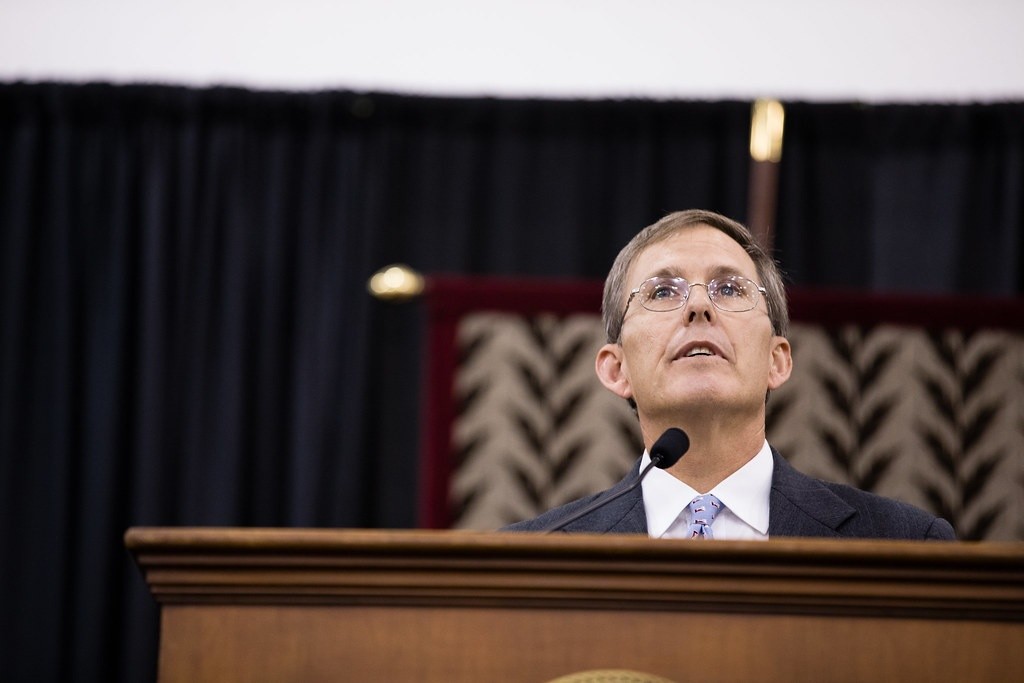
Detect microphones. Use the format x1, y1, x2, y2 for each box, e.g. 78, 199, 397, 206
548, 427, 690, 533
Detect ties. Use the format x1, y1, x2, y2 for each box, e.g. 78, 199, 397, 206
683, 494, 726, 539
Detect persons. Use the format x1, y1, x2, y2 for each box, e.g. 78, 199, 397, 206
497, 210, 956, 541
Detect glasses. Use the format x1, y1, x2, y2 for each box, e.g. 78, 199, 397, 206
616, 274, 772, 342
